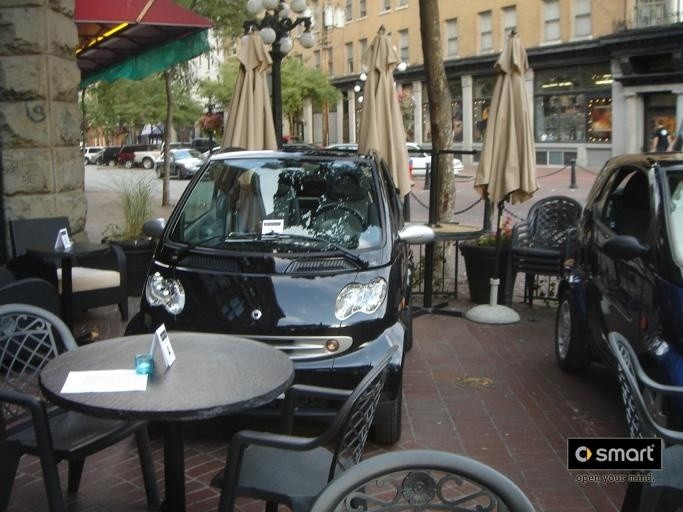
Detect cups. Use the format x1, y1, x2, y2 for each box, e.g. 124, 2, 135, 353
135, 354, 153, 373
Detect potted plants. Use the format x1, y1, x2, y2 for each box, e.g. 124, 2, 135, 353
101, 178, 157, 298
458, 212, 518, 306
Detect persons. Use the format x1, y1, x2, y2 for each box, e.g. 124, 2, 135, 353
649, 120, 671, 151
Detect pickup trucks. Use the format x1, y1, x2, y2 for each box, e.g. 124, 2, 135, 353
133, 146, 165, 169
133, 142, 167, 169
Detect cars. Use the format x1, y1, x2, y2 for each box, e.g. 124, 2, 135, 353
556, 154, 683, 439
123, 145, 435, 445
154, 149, 210, 179
407, 144, 462, 176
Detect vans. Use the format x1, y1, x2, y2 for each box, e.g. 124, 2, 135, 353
192, 139, 218, 151
115, 145, 157, 168
84, 146, 105, 165
91, 147, 121, 164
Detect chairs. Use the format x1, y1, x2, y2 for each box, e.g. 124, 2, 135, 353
9, 216, 128, 332
0, 304, 162, 512
305, 449, 537, 512
503, 196, 583, 308
210, 344, 401, 512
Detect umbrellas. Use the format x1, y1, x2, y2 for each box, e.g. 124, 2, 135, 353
221, 25, 277, 151
358, 26, 411, 207
464, 29, 541, 326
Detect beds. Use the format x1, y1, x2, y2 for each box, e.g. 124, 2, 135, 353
38, 331, 296, 512
